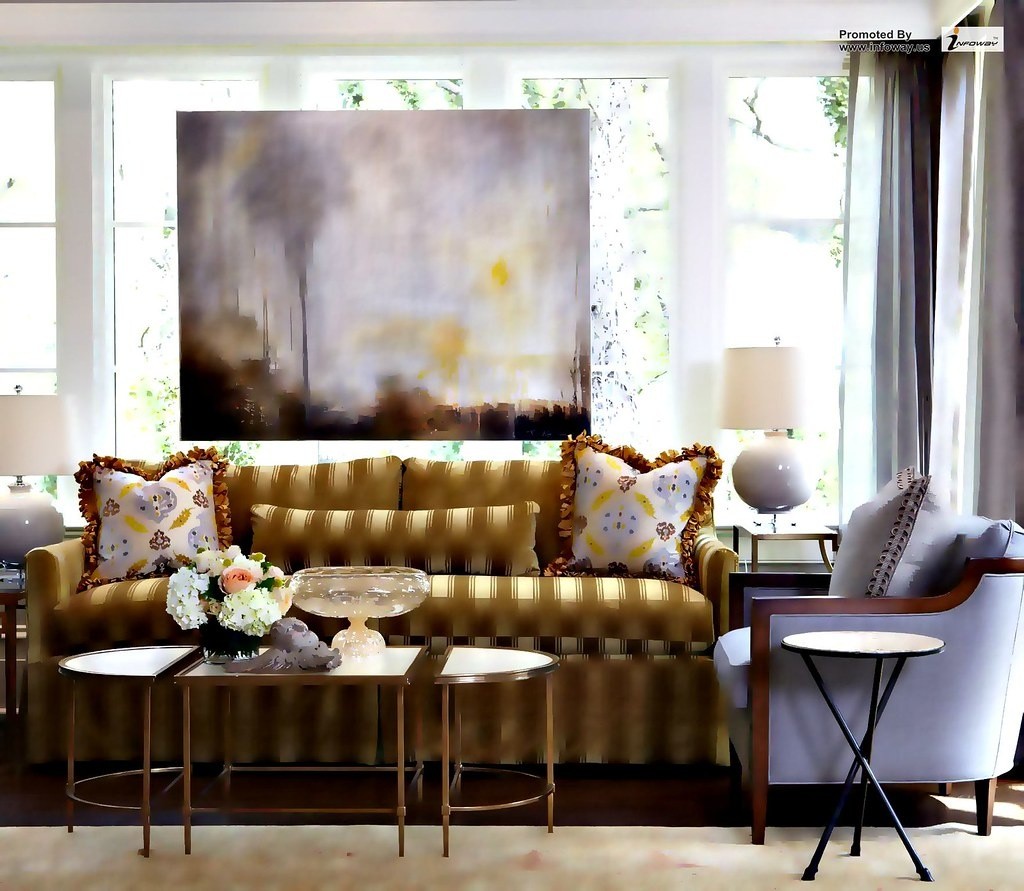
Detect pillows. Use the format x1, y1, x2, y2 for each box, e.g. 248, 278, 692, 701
830, 466, 956, 600
543, 429, 726, 590
74, 446, 232, 593
250, 500, 540, 576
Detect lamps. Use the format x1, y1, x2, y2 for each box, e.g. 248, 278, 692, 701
0, 385, 81, 589
714, 335, 839, 526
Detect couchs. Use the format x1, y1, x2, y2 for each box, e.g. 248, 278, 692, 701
711, 512, 1022, 846
25, 455, 738, 781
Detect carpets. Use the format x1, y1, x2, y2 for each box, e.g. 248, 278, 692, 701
0, 780, 1024, 891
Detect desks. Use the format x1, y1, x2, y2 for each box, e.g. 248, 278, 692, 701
1, 590, 28, 744
781, 628, 945, 882
434, 644, 560, 859
174, 643, 432, 858
57, 645, 201, 860
